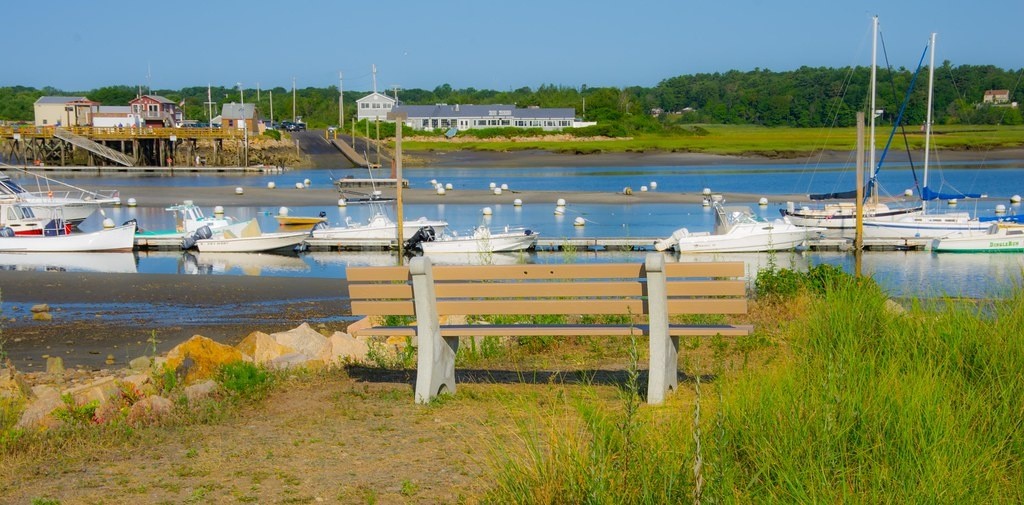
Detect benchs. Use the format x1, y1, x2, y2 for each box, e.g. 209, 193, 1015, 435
346, 251, 754, 404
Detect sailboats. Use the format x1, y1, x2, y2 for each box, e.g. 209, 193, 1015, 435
777, 13, 1024, 252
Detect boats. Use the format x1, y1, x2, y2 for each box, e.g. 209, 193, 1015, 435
179, 225, 309, 255
333, 178, 410, 207
132, 200, 264, 248
399, 223, 540, 257
0, 218, 138, 252
653, 200, 828, 254
0, 160, 121, 236
273, 211, 328, 225
305, 152, 449, 249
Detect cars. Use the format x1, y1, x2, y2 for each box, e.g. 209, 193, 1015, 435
280, 120, 292, 128
272, 123, 281, 130
327, 126, 338, 134
286, 121, 301, 132
